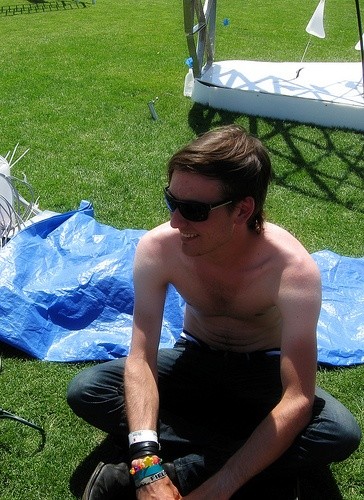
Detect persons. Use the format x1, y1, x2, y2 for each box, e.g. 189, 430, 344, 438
64, 123, 363, 499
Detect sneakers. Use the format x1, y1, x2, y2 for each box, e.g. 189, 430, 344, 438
79, 458, 132, 499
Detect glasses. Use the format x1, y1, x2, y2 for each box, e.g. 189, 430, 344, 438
160, 184, 242, 223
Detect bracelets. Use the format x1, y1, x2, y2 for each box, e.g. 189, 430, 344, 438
134, 469, 168, 488
130, 454, 162, 475
129, 441, 161, 460
131, 464, 164, 478
128, 429, 158, 448
